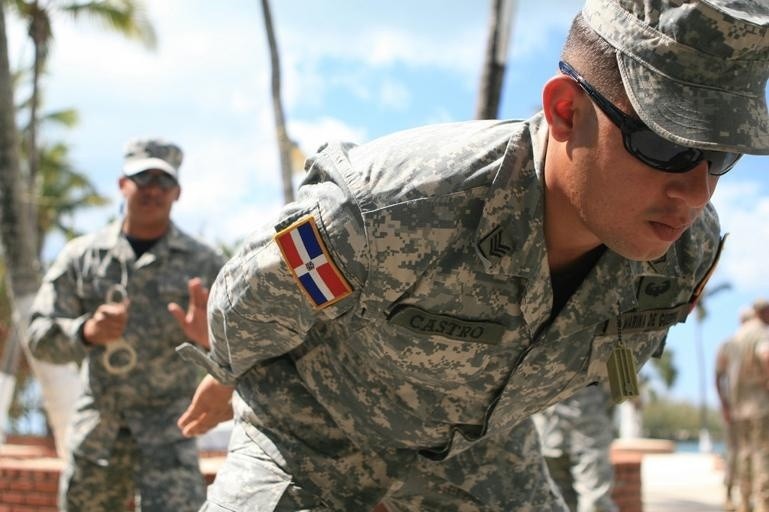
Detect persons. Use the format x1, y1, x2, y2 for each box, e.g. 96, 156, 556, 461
712, 301, 769, 512
177, 2, 768, 512
27, 137, 231, 512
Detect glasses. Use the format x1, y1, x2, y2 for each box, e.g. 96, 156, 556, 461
556, 60, 749, 177
129, 173, 177, 192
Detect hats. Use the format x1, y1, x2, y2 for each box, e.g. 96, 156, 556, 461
122, 135, 185, 188
581, 1, 769, 156
